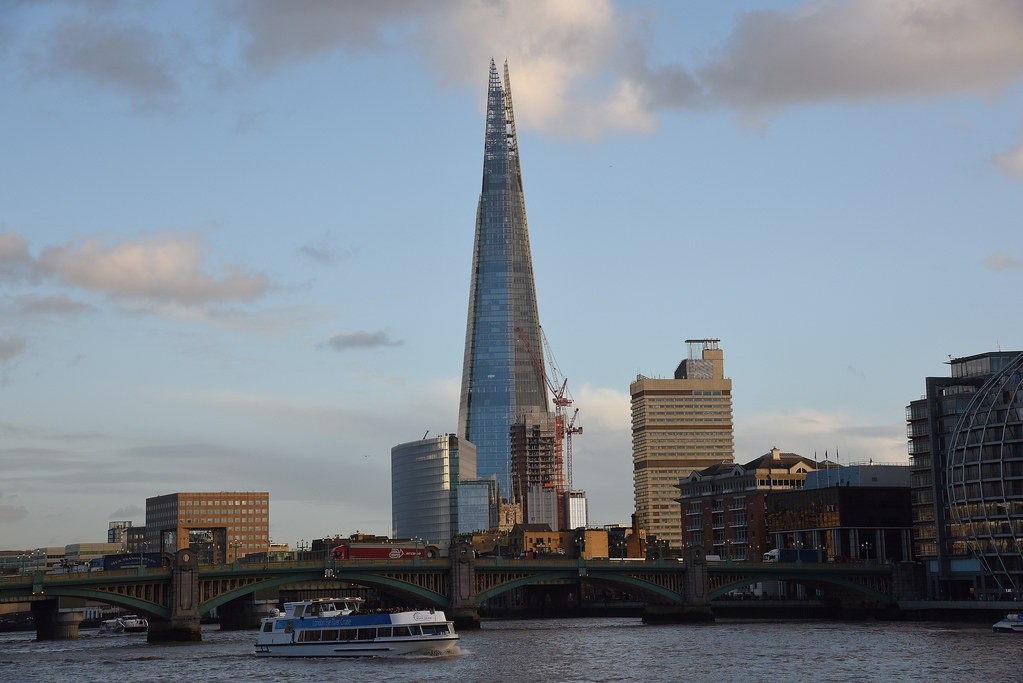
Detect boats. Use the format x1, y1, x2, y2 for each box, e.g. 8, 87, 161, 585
253, 596, 461, 657
992, 613, 1023, 632
98, 614, 149, 633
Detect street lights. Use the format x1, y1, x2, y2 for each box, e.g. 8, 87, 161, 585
322, 535, 334, 558
792, 540, 803, 564
18, 553, 31, 573
655, 537, 665, 559
575, 536, 586, 560
230, 540, 242, 562
862, 542, 872, 561
724, 538, 733, 557
296, 539, 308, 562
750, 545, 759, 561
411, 536, 422, 558
617, 541, 627, 561
493, 536, 504, 559
31, 549, 45, 574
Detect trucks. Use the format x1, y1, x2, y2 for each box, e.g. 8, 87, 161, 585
330, 541, 426, 560
87, 551, 161, 573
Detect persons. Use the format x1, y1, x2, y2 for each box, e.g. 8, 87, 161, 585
524, 547, 539, 560
335, 611, 344, 617
348, 605, 435, 616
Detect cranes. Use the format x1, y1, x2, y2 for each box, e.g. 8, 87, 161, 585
515, 324, 583, 531
